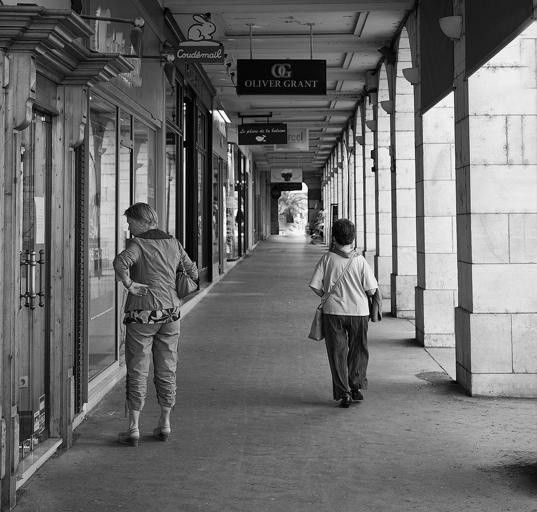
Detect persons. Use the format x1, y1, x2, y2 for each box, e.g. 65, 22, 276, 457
309, 217, 378, 407
310, 212, 324, 240
113, 202, 200, 448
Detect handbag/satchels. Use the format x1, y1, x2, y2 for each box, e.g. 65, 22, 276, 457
307, 302, 326, 342
175, 236, 199, 299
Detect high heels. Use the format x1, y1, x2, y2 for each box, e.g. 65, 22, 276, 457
152, 425, 171, 441
118, 428, 140, 447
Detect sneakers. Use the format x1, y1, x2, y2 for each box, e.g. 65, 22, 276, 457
341, 391, 353, 406
350, 384, 365, 401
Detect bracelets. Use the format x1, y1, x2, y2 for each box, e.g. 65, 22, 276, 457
125, 279, 134, 288
